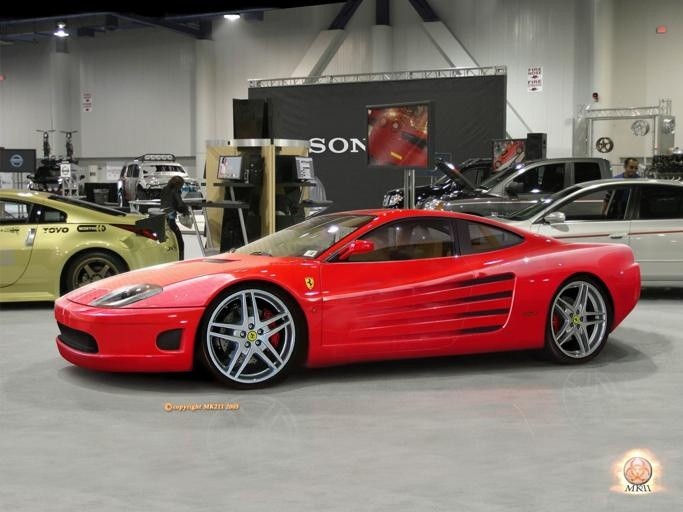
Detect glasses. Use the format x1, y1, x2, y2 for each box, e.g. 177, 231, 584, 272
630, 166, 638, 169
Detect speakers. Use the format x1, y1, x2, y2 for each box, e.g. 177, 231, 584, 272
526, 132, 548, 160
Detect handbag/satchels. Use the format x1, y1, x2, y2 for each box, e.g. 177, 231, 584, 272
178, 215, 194, 229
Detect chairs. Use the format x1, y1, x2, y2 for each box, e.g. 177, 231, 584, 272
388, 224, 431, 261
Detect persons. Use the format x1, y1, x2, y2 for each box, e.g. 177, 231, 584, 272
218, 158, 229, 177
160, 173, 192, 262
600, 158, 641, 219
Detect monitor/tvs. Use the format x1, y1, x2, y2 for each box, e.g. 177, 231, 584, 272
490, 138, 527, 174
217, 155, 244, 182
84, 182, 120, 207
1, 149, 37, 173
294, 156, 317, 183
364, 99, 436, 170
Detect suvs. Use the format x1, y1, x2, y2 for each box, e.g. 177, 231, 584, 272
120, 154, 204, 212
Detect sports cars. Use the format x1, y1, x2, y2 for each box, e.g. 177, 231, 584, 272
0, 188, 183, 306
54, 209, 641, 390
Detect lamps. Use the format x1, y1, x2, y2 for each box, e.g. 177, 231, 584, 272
55, 22, 69, 38
